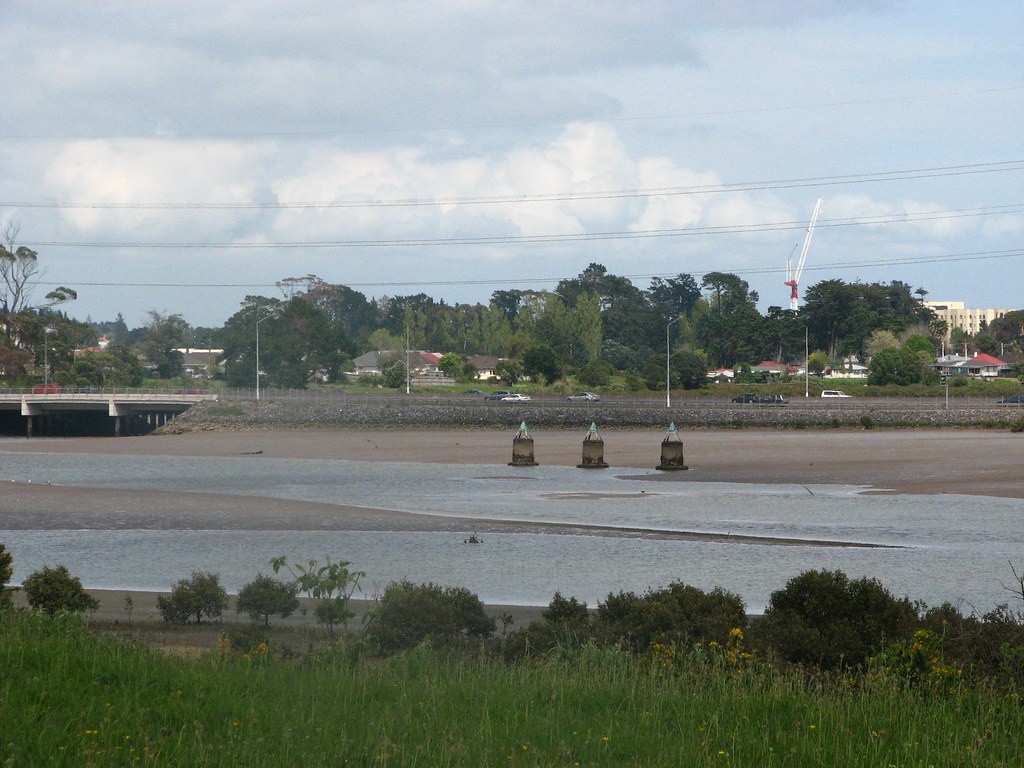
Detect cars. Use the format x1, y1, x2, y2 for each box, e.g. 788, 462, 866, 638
465, 389, 482, 394
566, 391, 601, 402
501, 393, 532, 404
759, 393, 789, 404
996, 394, 1024, 404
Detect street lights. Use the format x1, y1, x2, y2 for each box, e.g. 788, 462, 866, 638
666, 315, 684, 408
793, 318, 809, 397
389, 315, 410, 393
256, 313, 276, 400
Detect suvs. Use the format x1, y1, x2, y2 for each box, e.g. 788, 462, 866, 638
732, 393, 760, 403
484, 390, 513, 401
820, 390, 853, 398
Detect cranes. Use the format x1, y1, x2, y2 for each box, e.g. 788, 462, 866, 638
783, 196, 822, 311
